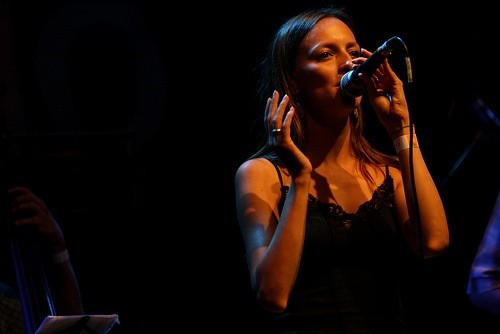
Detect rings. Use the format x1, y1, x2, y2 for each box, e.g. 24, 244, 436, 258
271, 128, 284, 136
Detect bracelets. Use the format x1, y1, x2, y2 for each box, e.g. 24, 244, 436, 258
393, 133, 421, 156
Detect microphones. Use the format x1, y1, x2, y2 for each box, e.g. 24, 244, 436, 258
339, 36, 398, 98
471, 97, 500, 137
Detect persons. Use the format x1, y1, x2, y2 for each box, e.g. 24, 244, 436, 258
463, 193, 500, 316
233, 8, 451, 334
0, 184, 87, 334
